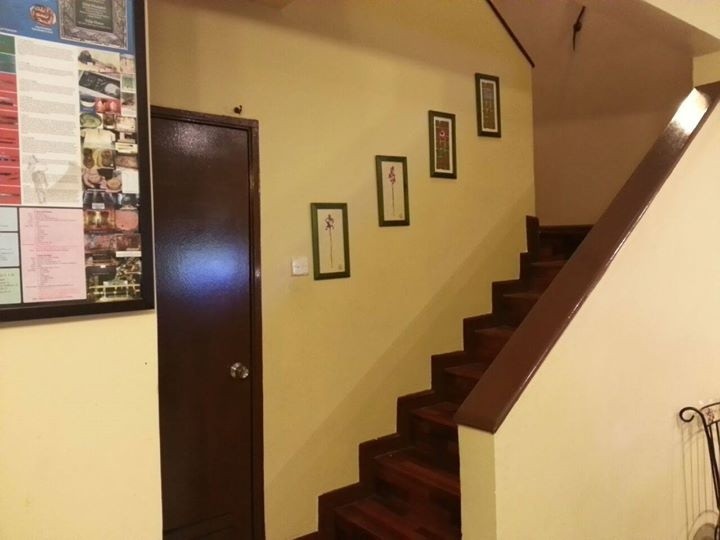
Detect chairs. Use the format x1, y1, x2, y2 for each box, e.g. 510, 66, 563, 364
677, 397, 720, 540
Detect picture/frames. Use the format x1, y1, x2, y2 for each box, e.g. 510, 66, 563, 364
375, 152, 415, 230
426, 107, 460, 180
309, 199, 353, 283
472, 69, 505, 136
